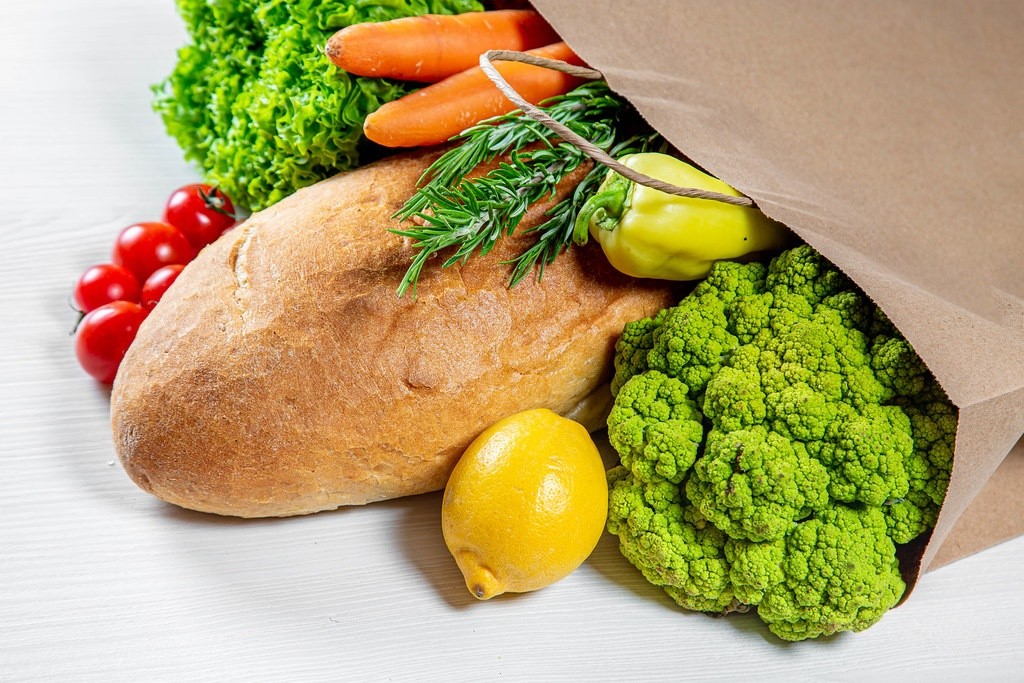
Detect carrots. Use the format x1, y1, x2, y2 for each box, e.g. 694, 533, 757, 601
330, 10, 594, 147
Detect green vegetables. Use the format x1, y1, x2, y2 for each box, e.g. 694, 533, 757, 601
390, 81, 674, 297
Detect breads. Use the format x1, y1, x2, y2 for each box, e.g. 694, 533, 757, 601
111, 144, 670, 517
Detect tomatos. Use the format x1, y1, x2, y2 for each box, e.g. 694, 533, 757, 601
74, 183, 239, 382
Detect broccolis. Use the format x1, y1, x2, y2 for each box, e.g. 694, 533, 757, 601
602, 243, 959, 642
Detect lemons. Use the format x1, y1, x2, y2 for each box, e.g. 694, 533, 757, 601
442, 407, 608, 601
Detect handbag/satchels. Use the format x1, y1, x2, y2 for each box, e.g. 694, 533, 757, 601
480, 1, 1024, 608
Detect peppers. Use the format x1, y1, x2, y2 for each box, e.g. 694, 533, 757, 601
574, 153, 791, 281
